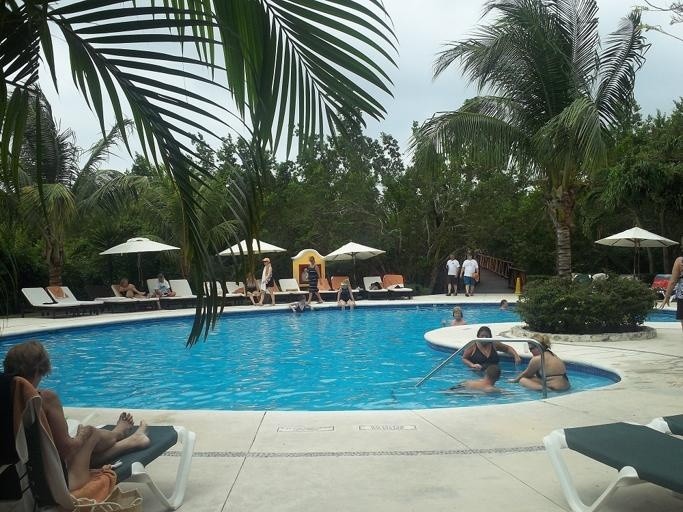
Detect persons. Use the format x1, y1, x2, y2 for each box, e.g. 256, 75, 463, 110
501, 332, 570, 393
459, 250, 478, 297
244, 271, 263, 306
446, 365, 515, 399
4, 340, 152, 490
118, 276, 145, 298
499, 298, 511, 310
335, 278, 355, 311
256, 258, 276, 306
304, 256, 324, 304
450, 305, 466, 325
473, 249, 480, 282
461, 325, 521, 378
150, 272, 176, 297
445, 253, 460, 296
659, 255, 683, 331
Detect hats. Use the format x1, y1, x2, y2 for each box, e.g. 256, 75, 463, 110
341, 279, 350, 285
262, 258, 270, 262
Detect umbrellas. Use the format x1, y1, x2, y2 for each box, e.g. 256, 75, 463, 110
98, 237, 180, 291
592, 226, 681, 277
323, 241, 386, 285
213, 237, 287, 280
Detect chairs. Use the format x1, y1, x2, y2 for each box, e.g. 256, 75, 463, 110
21, 274, 413, 319
650, 274, 673, 306
0, 372, 197, 512
544, 414, 683, 512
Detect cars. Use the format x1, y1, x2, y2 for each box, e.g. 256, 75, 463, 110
651, 274, 672, 288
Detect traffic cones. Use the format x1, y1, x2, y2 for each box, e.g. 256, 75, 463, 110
514, 277, 523, 294
